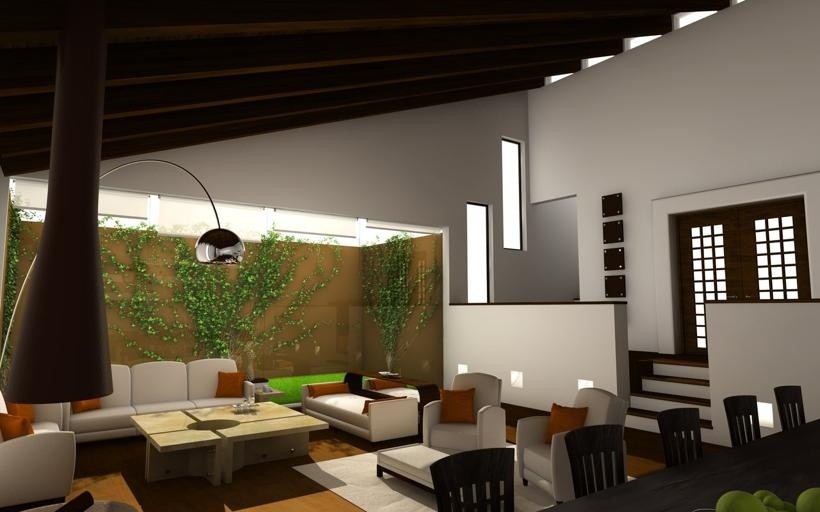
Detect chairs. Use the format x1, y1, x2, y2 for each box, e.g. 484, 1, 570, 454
428, 444, 517, 510
513, 387, 629, 502
419, 372, 508, 457
720, 394, 761, 450
773, 384, 806, 433
657, 407, 705, 469
564, 424, 627, 498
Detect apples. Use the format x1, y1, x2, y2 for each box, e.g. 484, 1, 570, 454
716, 488, 820, 512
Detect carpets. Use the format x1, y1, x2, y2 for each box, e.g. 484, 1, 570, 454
287, 446, 641, 511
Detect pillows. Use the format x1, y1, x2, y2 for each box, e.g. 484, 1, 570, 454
437, 387, 478, 425
0, 412, 34, 443
3, 396, 37, 424
70, 396, 103, 416
215, 371, 248, 399
544, 402, 589, 444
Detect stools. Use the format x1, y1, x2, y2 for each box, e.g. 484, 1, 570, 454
373, 441, 475, 502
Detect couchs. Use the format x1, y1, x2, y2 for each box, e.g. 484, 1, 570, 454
344, 366, 440, 412
0, 356, 257, 508
300, 381, 420, 444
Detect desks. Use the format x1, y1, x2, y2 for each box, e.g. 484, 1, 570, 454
533, 417, 820, 510
255, 386, 287, 403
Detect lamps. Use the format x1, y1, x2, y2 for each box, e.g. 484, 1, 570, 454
0, 158, 246, 383
